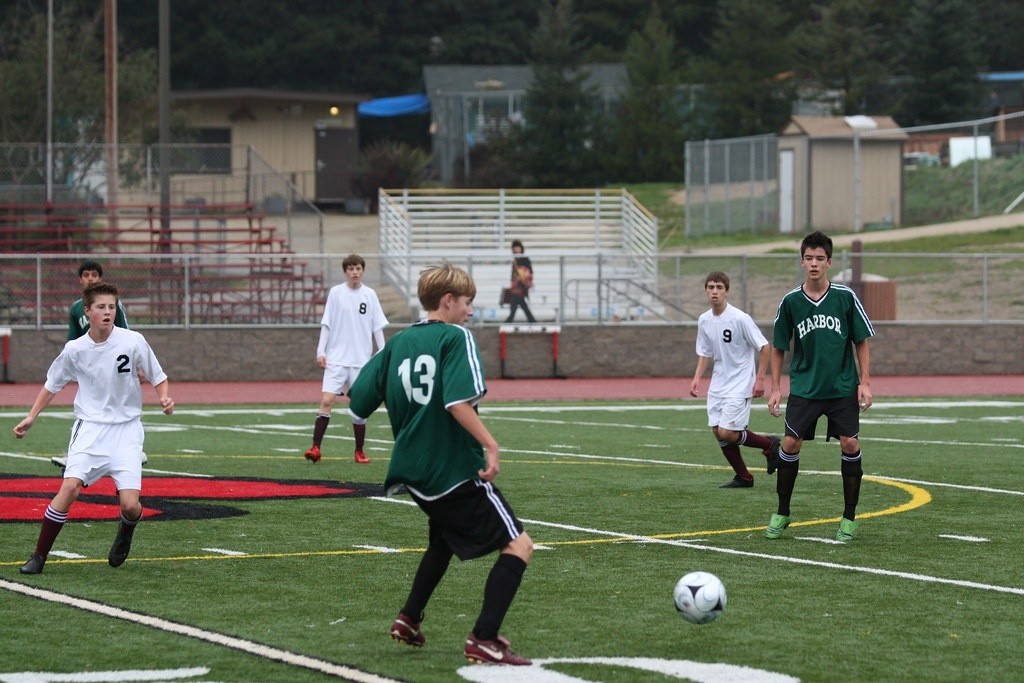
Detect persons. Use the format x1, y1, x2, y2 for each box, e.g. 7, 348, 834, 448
305, 253, 390, 465
13, 281, 175, 575
500, 239, 537, 323
765, 229, 876, 542
690, 271, 782, 490
49, 262, 148, 468
347, 262, 534, 666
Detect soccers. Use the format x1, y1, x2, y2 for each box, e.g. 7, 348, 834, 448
673, 572, 728, 624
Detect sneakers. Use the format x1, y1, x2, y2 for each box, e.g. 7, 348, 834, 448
464, 629, 532, 666
355, 449, 369, 463
52, 456, 67, 466
19, 555, 44, 574
761, 434, 782, 475
304, 446, 321, 463
763, 511, 791, 539
718, 473, 754, 488
836, 517, 858, 541
108, 523, 135, 567
389, 612, 425, 647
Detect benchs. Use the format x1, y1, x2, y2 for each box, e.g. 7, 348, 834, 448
0, 203, 333, 333
